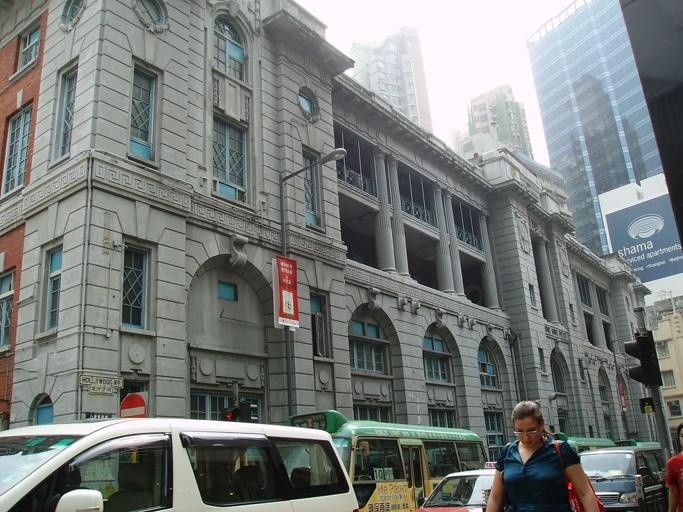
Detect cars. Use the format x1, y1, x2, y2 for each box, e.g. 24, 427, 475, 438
408, 460, 503, 512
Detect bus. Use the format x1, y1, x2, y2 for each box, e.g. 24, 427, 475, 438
284, 410, 491, 512
548, 431, 617, 455
613, 439, 661, 448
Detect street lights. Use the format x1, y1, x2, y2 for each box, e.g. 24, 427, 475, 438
278, 146, 347, 419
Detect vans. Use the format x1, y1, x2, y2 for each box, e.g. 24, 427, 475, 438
0, 416, 362, 512
577, 445, 669, 511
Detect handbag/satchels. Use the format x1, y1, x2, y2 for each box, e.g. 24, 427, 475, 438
567, 475, 605, 512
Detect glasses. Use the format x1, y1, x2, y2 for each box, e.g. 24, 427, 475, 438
513, 431, 537, 436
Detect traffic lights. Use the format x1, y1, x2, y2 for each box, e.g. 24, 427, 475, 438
622, 327, 663, 388
224, 406, 239, 421
641, 399, 654, 413
239, 400, 259, 423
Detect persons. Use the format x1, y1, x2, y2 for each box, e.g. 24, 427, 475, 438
666, 423, 683, 512
486, 400, 599, 512
411, 445, 460, 487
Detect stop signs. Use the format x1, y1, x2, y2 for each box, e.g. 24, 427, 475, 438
119, 393, 146, 418
